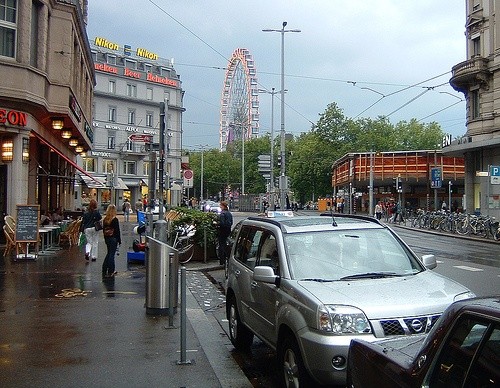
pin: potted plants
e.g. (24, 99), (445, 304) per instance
(165, 206), (218, 260)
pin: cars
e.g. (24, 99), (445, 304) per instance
(145, 200), (167, 215)
(199, 200), (229, 213)
(345, 293), (500, 388)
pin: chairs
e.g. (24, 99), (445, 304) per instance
(3, 216), (21, 256)
(58, 216), (83, 247)
(260, 238), (277, 258)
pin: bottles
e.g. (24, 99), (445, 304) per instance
(140, 232), (146, 244)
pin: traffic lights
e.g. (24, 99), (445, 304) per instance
(398, 181), (403, 193)
(166, 175), (174, 190)
(392, 178), (399, 192)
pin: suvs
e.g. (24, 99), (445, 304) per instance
(224, 209), (488, 388)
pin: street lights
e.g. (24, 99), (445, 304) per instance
(258, 21), (302, 209)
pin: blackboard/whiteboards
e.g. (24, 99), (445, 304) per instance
(14, 204), (41, 243)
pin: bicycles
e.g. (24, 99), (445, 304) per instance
(410, 208), (500, 241)
(166, 227), (196, 264)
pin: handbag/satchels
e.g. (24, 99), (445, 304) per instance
(374, 214), (377, 218)
(122, 203), (126, 211)
(78, 232), (82, 246)
(94, 221), (103, 231)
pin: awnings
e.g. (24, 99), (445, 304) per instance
(81, 175), (129, 190)
(142, 178), (182, 191)
(32, 129), (106, 188)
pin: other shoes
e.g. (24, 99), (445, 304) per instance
(92, 259), (96, 261)
(102, 274), (113, 281)
(85, 253), (89, 262)
(108, 271), (119, 275)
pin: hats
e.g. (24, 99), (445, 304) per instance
(221, 202), (228, 206)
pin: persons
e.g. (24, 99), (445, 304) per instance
(182, 195), (199, 209)
(83, 200), (102, 262)
(134, 195), (148, 222)
(102, 204), (121, 278)
(375, 199), (446, 224)
(218, 201), (233, 264)
(263, 194), (344, 215)
(122, 199), (130, 222)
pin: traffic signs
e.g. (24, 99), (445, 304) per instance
(128, 134), (153, 143)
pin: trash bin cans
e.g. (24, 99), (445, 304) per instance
(137, 210), (145, 226)
(145, 236), (180, 315)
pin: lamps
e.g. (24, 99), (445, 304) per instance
(61, 128), (72, 139)
(68, 136), (79, 146)
(22, 138), (30, 164)
(50, 116), (64, 129)
(2, 136), (14, 163)
(81, 149), (88, 156)
(74, 144), (84, 154)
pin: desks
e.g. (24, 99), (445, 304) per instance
(30, 224), (63, 255)
